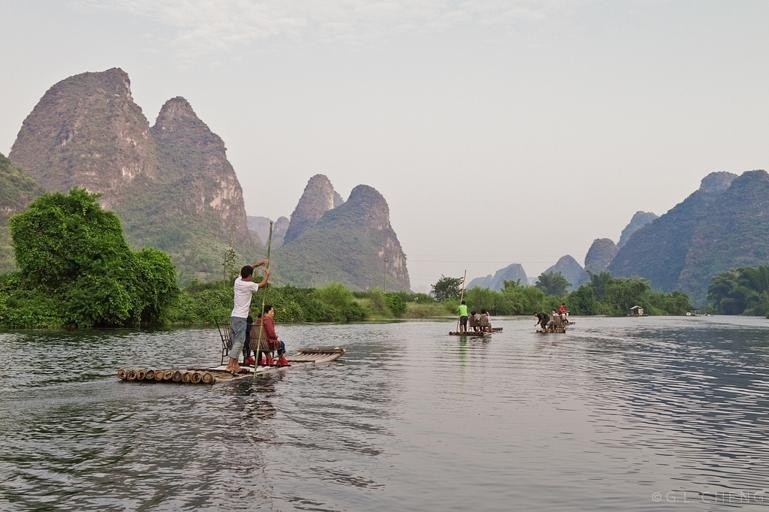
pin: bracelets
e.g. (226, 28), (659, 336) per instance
(254, 262), (259, 267)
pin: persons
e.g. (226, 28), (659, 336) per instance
(456, 300), (469, 333)
(224, 313), (252, 366)
(226, 257), (271, 373)
(546, 309), (560, 331)
(253, 306), (288, 366)
(481, 310), (493, 332)
(558, 303), (570, 321)
(533, 311), (550, 334)
(468, 310), (483, 333)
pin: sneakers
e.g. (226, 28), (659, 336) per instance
(244, 357), (286, 366)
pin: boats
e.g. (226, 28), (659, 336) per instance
(449, 327), (503, 338)
(117, 345), (346, 387)
(536, 321), (576, 333)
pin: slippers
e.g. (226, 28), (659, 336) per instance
(235, 368), (250, 374)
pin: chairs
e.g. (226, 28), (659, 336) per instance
(218, 323), (282, 369)
